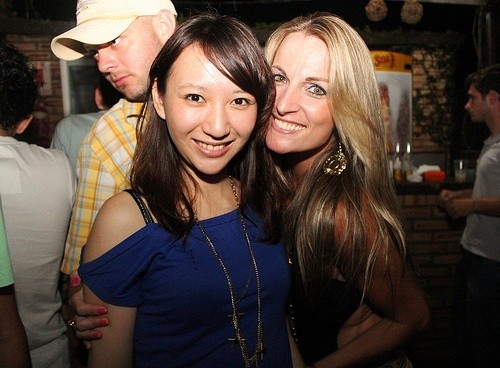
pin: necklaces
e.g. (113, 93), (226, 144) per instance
(187, 174), (264, 365)
(289, 296), (300, 348)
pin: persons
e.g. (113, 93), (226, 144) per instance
(0, 44), (77, 368)
(78, 13), (303, 368)
(67, 13), (430, 368)
(50, 0), (177, 322)
(439, 71), (500, 368)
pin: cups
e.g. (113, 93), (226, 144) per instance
(453, 160), (469, 183)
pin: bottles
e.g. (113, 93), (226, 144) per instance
(394, 157), (401, 181)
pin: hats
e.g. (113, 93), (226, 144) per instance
(50, 0), (178, 62)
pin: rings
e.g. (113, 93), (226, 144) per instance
(68, 320), (76, 331)
